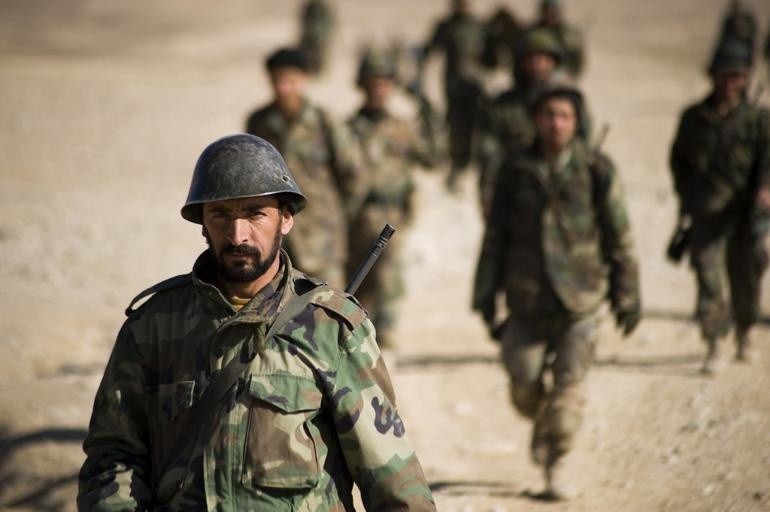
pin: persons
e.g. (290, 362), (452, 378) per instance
(77, 134), (438, 512)
(417, 0), (642, 502)
(664, 2), (770, 376)
(247, 1), (448, 507)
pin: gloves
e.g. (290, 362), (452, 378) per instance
(614, 304), (640, 339)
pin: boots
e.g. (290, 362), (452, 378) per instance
(736, 326), (758, 363)
(701, 336), (734, 377)
(543, 455), (580, 501)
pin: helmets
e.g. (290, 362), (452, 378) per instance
(526, 76), (584, 118)
(181, 133), (308, 226)
